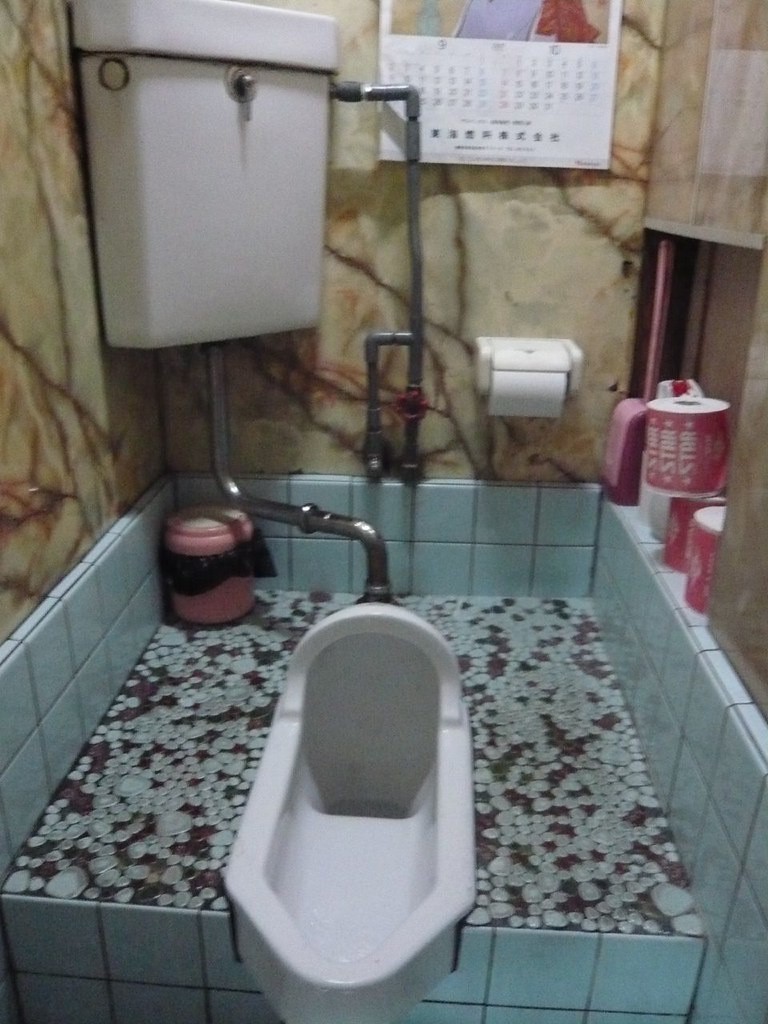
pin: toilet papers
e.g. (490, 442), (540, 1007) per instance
(643, 394), (727, 615)
(488, 369), (570, 421)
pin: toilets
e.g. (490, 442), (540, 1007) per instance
(225, 597), (478, 1024)
